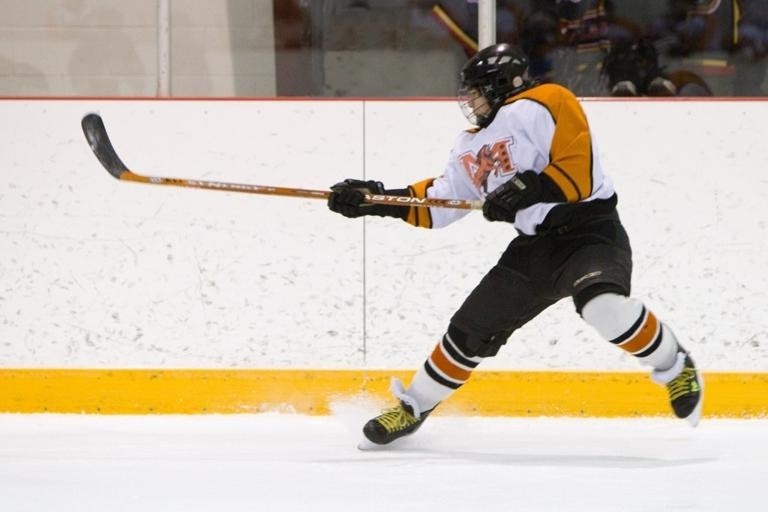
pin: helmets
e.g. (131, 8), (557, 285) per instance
(456, 43), (533, 128)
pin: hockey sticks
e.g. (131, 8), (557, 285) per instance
(81, 113), (484, 210)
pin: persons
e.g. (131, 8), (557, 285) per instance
(462, 0), (768, 98)
(327, 42), (701, 445)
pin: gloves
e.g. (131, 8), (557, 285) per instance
(328, 179), (411, 221)
(482, 172), (566, 223)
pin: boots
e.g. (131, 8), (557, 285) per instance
(362, 376), (441, 443)
(652, 344), (701, 419)
